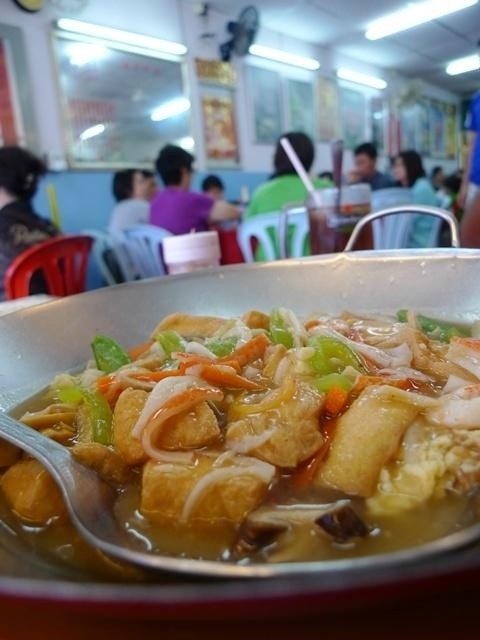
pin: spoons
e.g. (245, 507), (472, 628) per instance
(0, 409), (479, 580)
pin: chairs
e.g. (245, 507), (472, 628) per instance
(5, 233), (94, 300)
(124, 223), (175, 277)
(94, 223), (132, 285)
(371, 189), (414, 251)
(234, 205), (313, 262)
(428, 194), (452, 248)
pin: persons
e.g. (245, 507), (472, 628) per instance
(0, 146), (63, 302)
(348, 143), (393, 190)
(442, 89), (480, 248)
(150, 145), (244, 235)
(203, 176), (223, 200)
(391, 150), (442, 248)
(432, 167), (443, 190)
(108, 169), (160, 236)
(240, 132), (337, 262)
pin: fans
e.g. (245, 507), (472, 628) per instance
(218, 6), (259, 61)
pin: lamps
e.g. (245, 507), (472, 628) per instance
(248, 44), (320, 70)
(337, 69), (387, 90)
(361, 0), (479, 41)
(444, 54), (480, 76)
(55, 18), (189, 56)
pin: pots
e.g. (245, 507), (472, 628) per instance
(0, 204), (480, 618)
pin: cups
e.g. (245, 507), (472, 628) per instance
(306, 183), (374, 256)
(160, 231), (220, 276)
